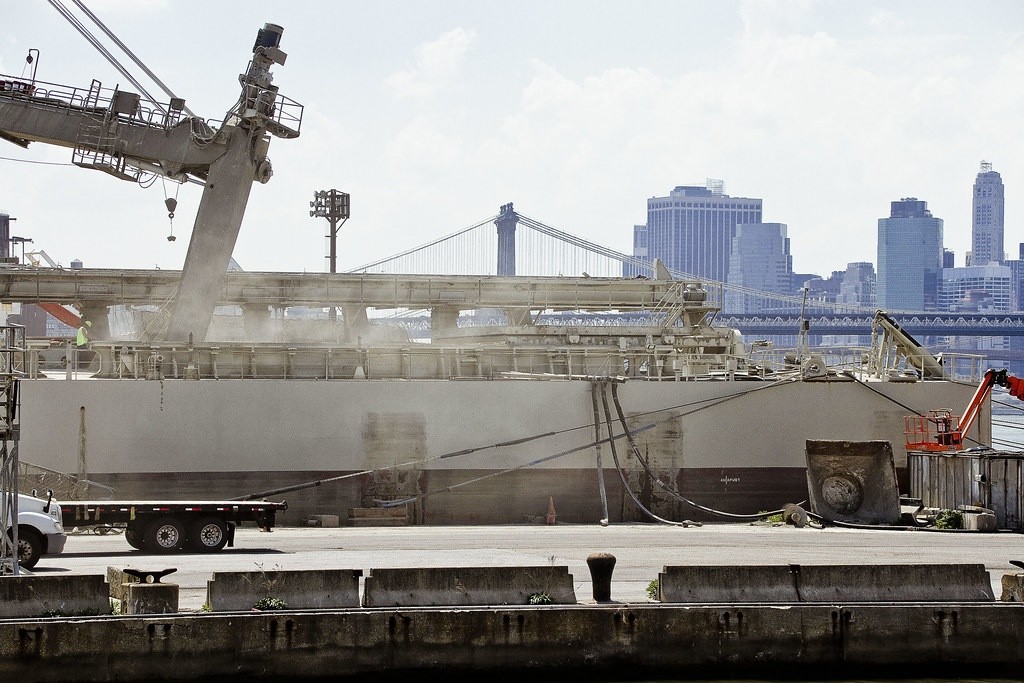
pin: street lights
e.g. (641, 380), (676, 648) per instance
(799, 287), (810, 323)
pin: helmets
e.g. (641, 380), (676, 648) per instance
(85, 321), (91, 327)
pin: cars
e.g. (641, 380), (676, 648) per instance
(0, 486), (70, 572)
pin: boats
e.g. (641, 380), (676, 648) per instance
(1, 2), (994, 523)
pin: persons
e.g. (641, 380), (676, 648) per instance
(76, 320), (93, 369)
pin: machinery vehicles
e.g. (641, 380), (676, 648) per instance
(901, 366), (1023, 451)
